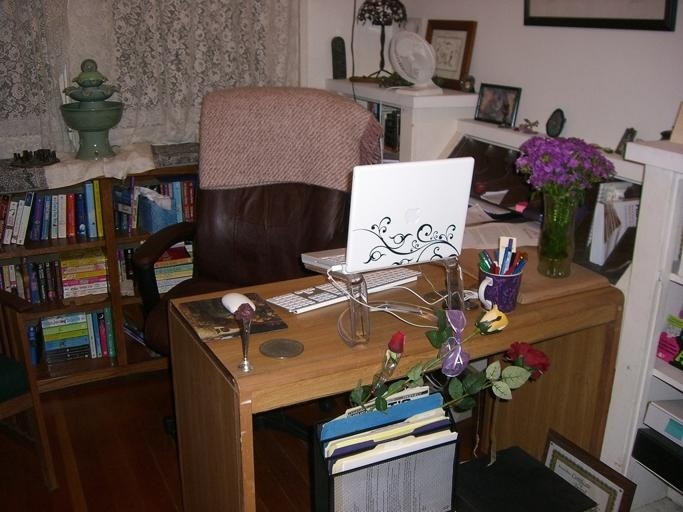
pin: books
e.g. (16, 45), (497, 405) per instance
(586, 183), (640, 266)
(0, 179), (195, 363)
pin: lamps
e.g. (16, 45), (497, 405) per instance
(350, 0), (408, 84)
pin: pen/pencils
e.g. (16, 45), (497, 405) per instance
(475, 239), (529, 293)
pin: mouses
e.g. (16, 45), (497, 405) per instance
(220, 291), (257, 318)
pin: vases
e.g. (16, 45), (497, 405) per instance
(536, 193), (576, 279)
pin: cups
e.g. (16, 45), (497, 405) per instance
(478, 262), (522, 314)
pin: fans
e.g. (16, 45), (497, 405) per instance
(388, 29), (444, 96)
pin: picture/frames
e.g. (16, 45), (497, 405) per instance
(524, 0), (678, 31)
(475, 82), (522, 128)
(425, 19), (478, 91)
(542, 427), (637, 512)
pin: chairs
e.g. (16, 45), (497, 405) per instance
(130, 86), (385, 443)
(0, 288), (60, 491)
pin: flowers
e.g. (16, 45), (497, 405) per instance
(515, 133), (618, 277)
(441, 341), (550, 410)
(348, 304), (509, 415)
(348, 329), (407, 412)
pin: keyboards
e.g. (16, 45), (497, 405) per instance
(266, 267), (421, 314)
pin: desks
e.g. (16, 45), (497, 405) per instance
(166, 245), (625, 512)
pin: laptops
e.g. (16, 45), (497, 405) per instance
(301, 155), (476, 278)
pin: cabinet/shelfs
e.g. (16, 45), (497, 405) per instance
(326, 79), (479, 163)
(0, 142), (198, 396)
(600, 141), (682, 512)
